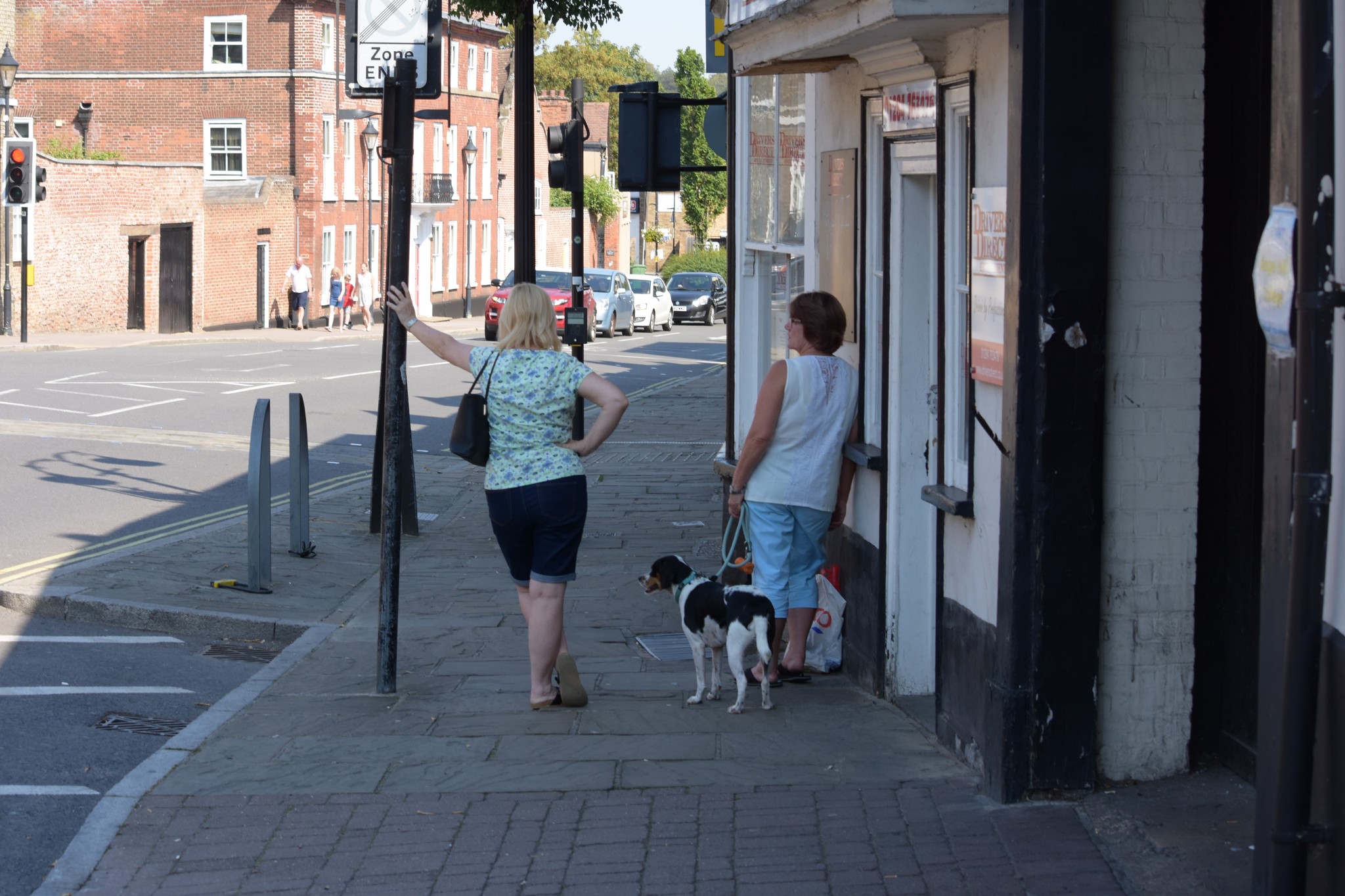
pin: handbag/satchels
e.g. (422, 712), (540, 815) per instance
(343, 287), (347, 296)
(782, 574), (847, 673)
(449, 347), (503, 467)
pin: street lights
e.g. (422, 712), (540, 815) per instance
(461, 135), (478, 319)
(361, 117), (380, 325)
(0, 42), (19, 337)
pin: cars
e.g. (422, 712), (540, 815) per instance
(571, 269), (636, 336)
(665, 272), (729, 326)
(484, 269), (597, 343)
(607, 275), (673, 333)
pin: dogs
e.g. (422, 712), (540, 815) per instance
(636, 553), (778, 715)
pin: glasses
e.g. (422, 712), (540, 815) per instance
(789, 318), (802, 327)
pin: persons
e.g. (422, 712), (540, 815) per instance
(384, 280), (629, 705)
(728, 289), (858, 686)
(281, 256), (375, 332)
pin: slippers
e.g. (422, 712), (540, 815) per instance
(776, 663), (811, 681)
(734, 667), (783, 687)
(530, 686), (564, 709)
(554, 652), (588, 707)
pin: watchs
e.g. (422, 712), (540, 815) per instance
(406, 317), (417, 331)
(729, 484), (745, 494)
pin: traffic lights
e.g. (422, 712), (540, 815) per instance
(2, 137), (36, 208)
(36, 165), (46, 204)
(546, 119), (576, 191)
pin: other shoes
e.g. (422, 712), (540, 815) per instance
(340, 327), (344, 333)
(348, 322), (353, 329)
(342, 325), (349, 330)
(325, 326), (332, 332)
(367, 324), (371, 331)
(362, 328), (367, 331)
(294, 325), (304, 331)
